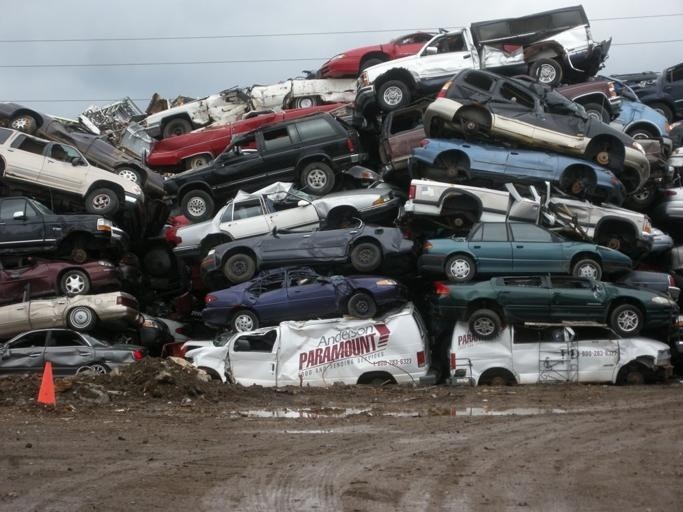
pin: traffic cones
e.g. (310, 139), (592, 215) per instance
(37, 362), (56, 407)
